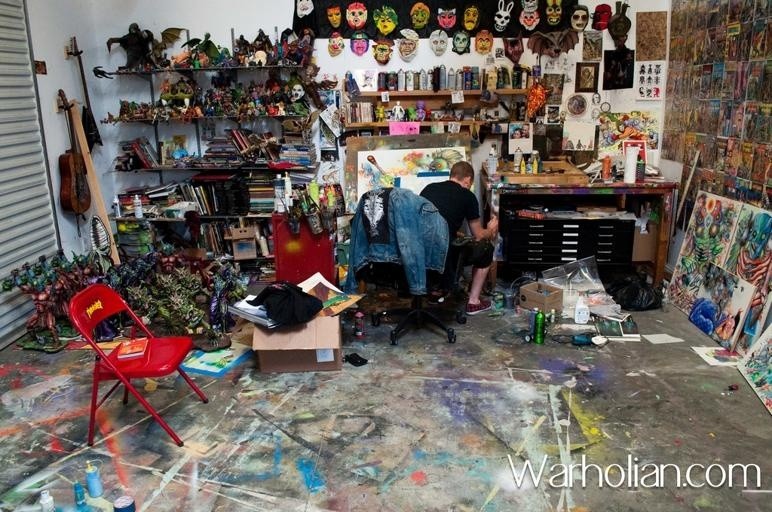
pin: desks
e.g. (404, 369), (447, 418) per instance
(479, 170), (681, 290)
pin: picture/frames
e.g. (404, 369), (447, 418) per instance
(575, 62), (599, 92)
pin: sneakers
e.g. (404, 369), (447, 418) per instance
(428, 288), (451, 304)
(465, 300), (491, 315)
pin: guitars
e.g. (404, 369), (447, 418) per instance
(58, 89), (90, 215)
(70, 36), (103, 153)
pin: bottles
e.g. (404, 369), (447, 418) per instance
(575, 291), (590, 324)
(521, 146), (542, 173)
(354, 312), (365, 337)
(488, 144), (498, 179)
(636, 159), (646, 181)
(602, 155), (611, 178)
(310, 180), (335, 207)
(519, 102), (526, 120)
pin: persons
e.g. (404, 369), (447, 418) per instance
(611, 120), (657, 149)
(3, 239), (248, 354)
(512, 124), (529, 139)
(93, 23), (338, 126)
(420, 162), (497, 315)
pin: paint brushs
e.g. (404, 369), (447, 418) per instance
(295, 187), (322, 214)
(277, 191), (290, 218)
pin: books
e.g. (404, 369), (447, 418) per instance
(117, 337), (148, 359)
(118, 128), (316, 286)
(346, 101), (376, 124)
(576, 206), (627, 217)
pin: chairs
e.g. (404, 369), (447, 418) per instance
(68, 285), (209, 447)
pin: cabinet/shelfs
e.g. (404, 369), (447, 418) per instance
(336, 90), (553, 155)
(110, 64), (323, 281)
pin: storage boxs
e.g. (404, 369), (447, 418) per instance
(252, 314), (343, 372)
(520, 281), (563, 315)
